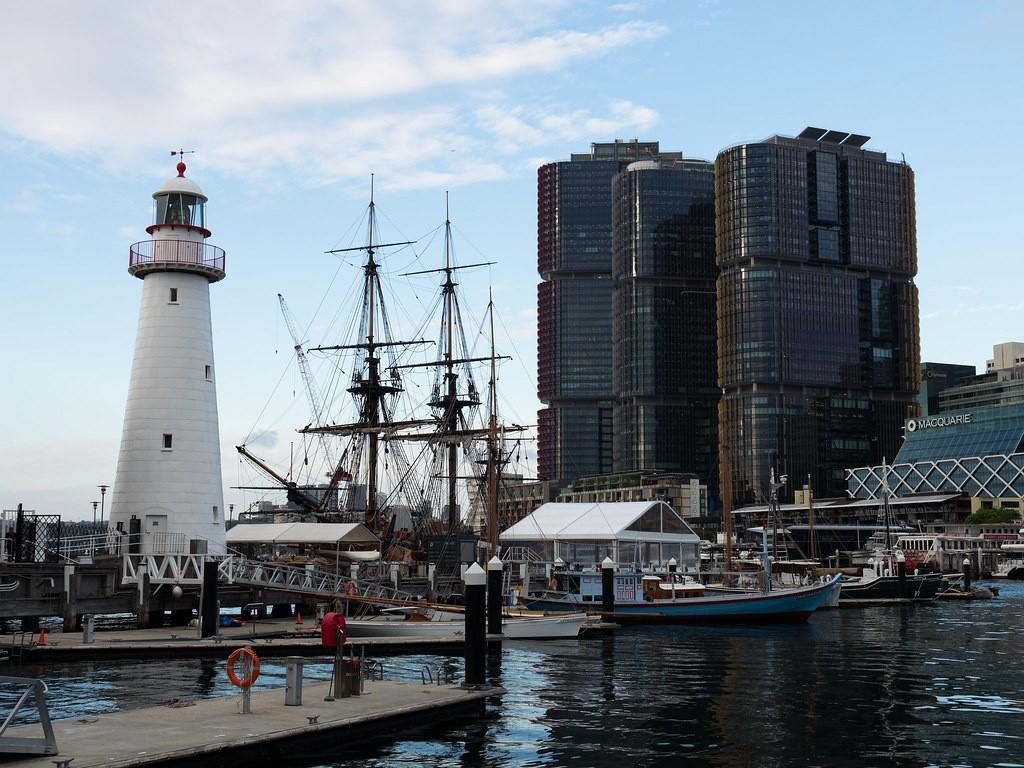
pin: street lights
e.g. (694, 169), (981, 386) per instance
(91, 501), (100, 523)
(97, 485), (110, 521)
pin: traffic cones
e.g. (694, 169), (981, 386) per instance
(36, 629), (47, 646)
(296, 612), (304, 625)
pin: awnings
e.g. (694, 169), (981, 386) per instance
(729, 493), (963, 515)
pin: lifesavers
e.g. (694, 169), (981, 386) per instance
(346, 582), (356, 595)
(644, 595), (653, 602)
(551, 579), (557, 590)
(226, 648), (260, 688)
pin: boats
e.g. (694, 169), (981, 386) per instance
(499, 498), (843, 628)
(705, 457), (942, 607)
(341, 611), (585, 644)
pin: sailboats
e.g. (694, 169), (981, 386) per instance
(241, 175), (539, 601)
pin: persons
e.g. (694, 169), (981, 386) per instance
(5, 526), (17, 562)
